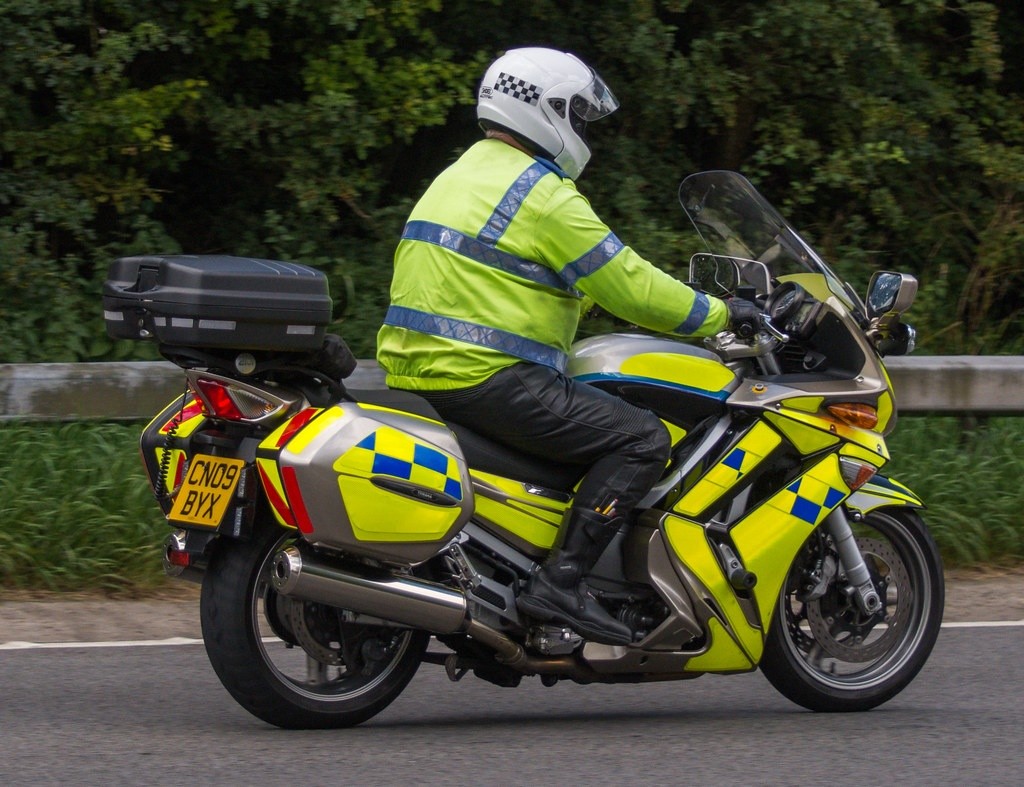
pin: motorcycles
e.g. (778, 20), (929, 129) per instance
(100, 170), (947, 730)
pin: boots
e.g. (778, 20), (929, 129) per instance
(515, 505), (633, 646)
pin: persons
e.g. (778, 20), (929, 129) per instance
(377, 48), (761, 645)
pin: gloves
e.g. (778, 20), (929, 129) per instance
(724, 296), (765, 341)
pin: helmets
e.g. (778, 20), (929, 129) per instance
(475, 47), (593, 183)
